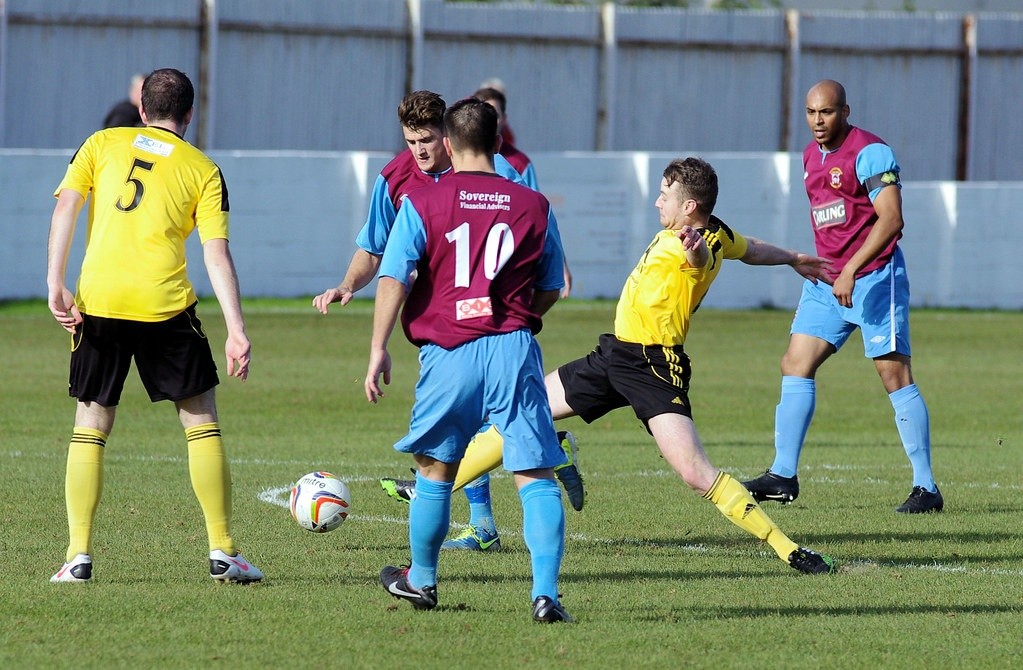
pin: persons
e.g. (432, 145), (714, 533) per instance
(380, 157), (834, 573)
(46, 67), (263, 588)
(103, 73), (147, 127)
(743, 79), (943, 516)
(313, 79), (594, 622)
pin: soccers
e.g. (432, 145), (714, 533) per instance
(288, 471), (352, 533)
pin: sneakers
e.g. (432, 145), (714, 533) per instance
(50, 554), (92, 583)
(210, 550), (263, 586)
(896, 484), (943, 513)
(381, 557), (438, 612)
(531, 594), (573, 624)
(553, 430), (584, 511)
(739, 469), (799, 502)
(380, 467), (418, 505)
(788, 548), (833, 576)
(439, 524), (502, 553)
